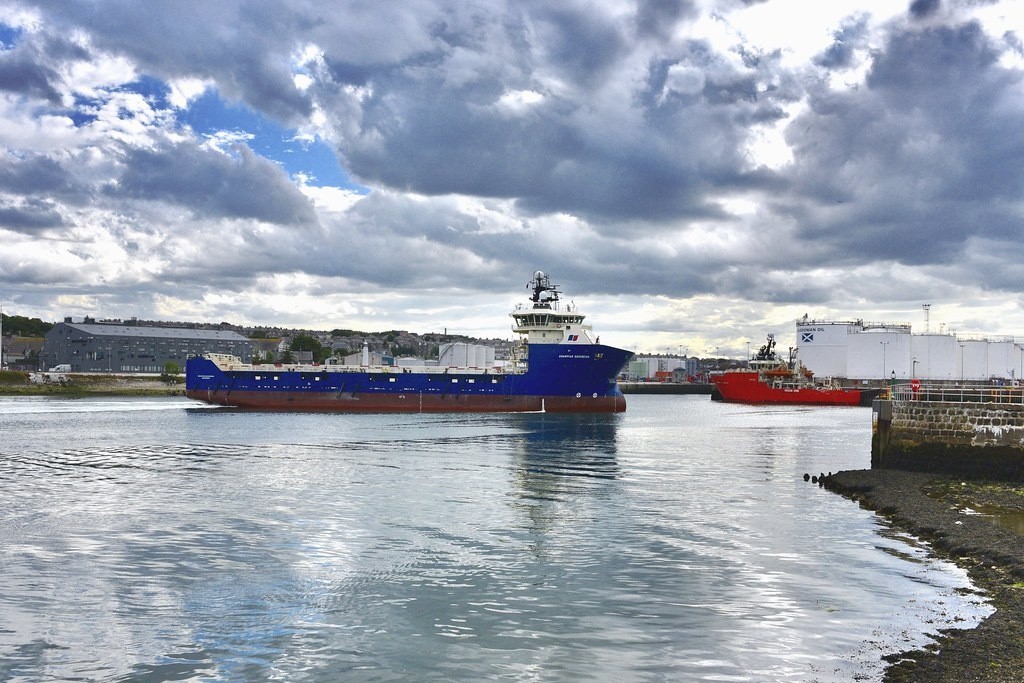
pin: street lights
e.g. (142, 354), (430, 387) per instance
(685, 347), (689, 358)
(746, 341), (752, 369)
(678, 344), (684, 359)
(667, 347), (670, 358)
(880, 340), (890, 390)
(715, 346), (720, 373)
(913, 361), (917, 382)
(959, 344), (967, 401)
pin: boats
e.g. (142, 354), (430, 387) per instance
(704, 332), (863, 406)
(184, 268), (636, 414)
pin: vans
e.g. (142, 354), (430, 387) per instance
(49, 363), (71, 374)
